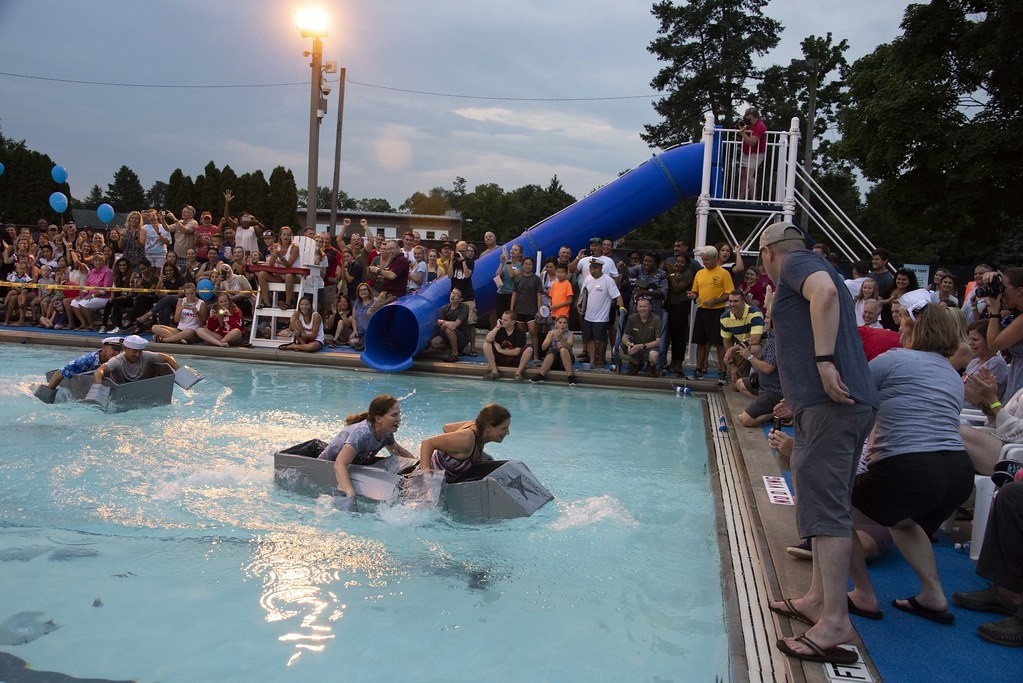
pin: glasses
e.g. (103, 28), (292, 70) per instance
(209, 246), (218, 250)
(907, 300), (931, 321)
(638, 296), (648, 300)
(451, 291), (461, 298)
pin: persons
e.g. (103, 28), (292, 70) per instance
(739, 107), (766, 204)
(0, 189), (477, 362)
(48, 335), (180, 390)
(479, 232), (793, 428)
(317, 395), (512, 496)
(756, 222), (1022, 663)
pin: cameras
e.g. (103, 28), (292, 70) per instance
(739, 118), (752, 127)
(975, 275), (1004, 299)
(453, 252), (460, 259)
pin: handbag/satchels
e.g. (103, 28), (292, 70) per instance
(493, 276), (503, 287)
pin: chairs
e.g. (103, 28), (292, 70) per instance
(939, 408), (1001, 560)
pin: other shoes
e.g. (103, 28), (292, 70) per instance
(786, 538), (812, 558)
(441, 349), (727, 385)
(953, 585), (1019, 615)
(4, 300), (363, 352)
(977, 613), (1023, 645)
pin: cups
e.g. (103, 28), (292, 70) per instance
(225, 247), (231, 258)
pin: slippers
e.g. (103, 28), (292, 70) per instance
(769, 599), (815, 626)
(846, 595), (884, 620)
(776, 633), (859, 664)
(893, 596), (955, 623)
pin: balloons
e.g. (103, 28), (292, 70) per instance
(197, 279), (215, 302)
(51, 165), (68, 184)
(49, 192), (68, 213)
(97, 203), (114, 224)
(0, 163), (4, 175)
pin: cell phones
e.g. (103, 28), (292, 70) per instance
(773, 416), (782, 433)
(242, 217), (251, 221)
(225, 247), (231, 254)
(134, 272), (140, 280)
(162, 211), (166, 216)
(503, 246), (507, 254)
(186, 304), (194, 309)
(213, 308), (225, 314)
(222, 271), (227, 281)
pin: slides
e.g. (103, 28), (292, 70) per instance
(361, 143), (708, 372)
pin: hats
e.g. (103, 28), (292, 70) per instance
(260, 230), (275, 239)
(47, 224), (59, 233)
(441, 242), (453, 250)
(760, 222), (805, 256)
(101, 337), (124, 350)
(200, 212), (211, 220)
(589, 258), (604, 267)
(590, 237), (602, 246)
(183, 204), (196, 214)
(124, 335), (148, 350)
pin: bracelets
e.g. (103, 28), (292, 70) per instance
(643, 344), (646, 350)
(377, 268), (381, 274)
(92, 294), (96, 298)
(299, 332), (301, 337)
(120, 236), (124, 239)
(133, 237), (138, 240)
(747, 354), (753, 361)
(990, 401), (1001, 410)
(280, 256), (284, 260)
(496, 325), (500, 330)
(989, 313), (1001, 318)
(506, 261), (512, 264)
(814, 355), (835, 362)
(174, 219), (178, 222)
(157, 232), (161, 235)
